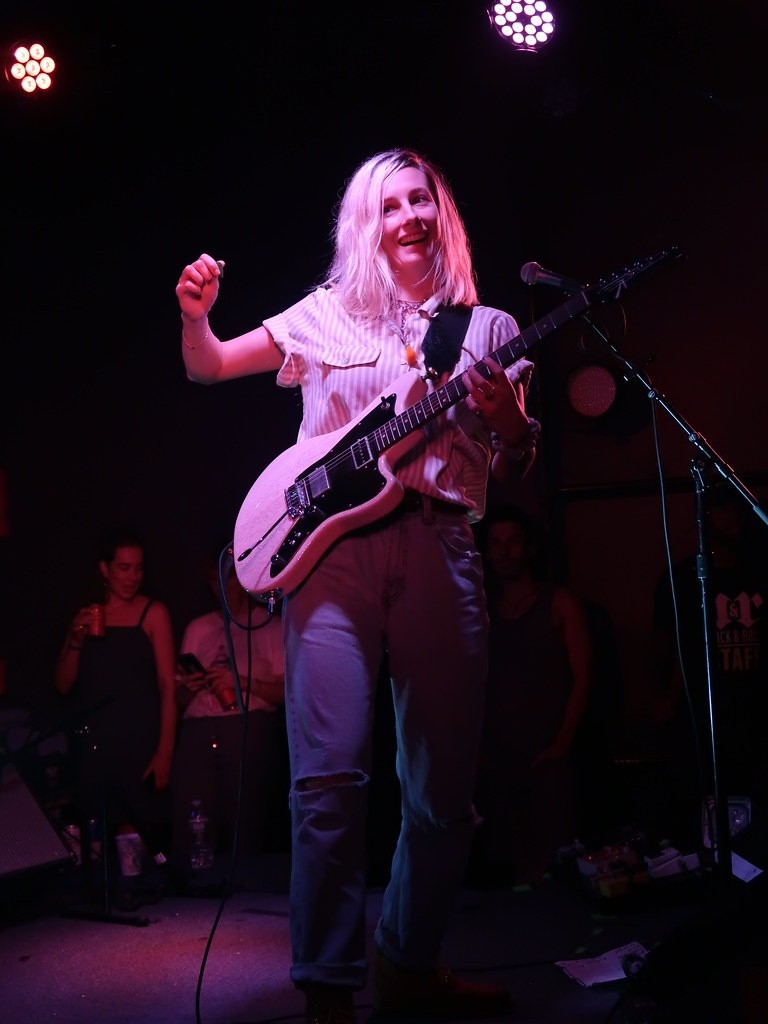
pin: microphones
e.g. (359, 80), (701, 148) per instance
(522, 262), (621, 304)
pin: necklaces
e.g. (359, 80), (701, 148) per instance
(387, 294), (428, 334)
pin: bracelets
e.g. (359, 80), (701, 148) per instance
(61, 637), (78, 650)
(182, 317), (209, 349)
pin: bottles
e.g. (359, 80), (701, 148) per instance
(188, 799), (209, 850)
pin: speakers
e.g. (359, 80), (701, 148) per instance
(0, 747), (79, 931)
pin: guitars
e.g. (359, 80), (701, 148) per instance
(232, 243), (690, 605)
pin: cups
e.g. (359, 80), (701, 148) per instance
(66, 826), (82, 866)
(115, 834), (144, 877)
(208, 680), (237, 713)
(87, 604), (105, 638)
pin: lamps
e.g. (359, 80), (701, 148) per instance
(565, 361), (653, 432)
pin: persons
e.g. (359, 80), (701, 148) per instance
(55, 482), (768, 997)
(174, 149), (543, 1024)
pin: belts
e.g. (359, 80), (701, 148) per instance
(389, 486), (473, 519)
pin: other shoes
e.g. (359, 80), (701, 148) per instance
(377, 959), (449, 1024)
(298, 984), (359, 1024)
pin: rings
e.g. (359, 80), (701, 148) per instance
(486, 386), (497, 399)
